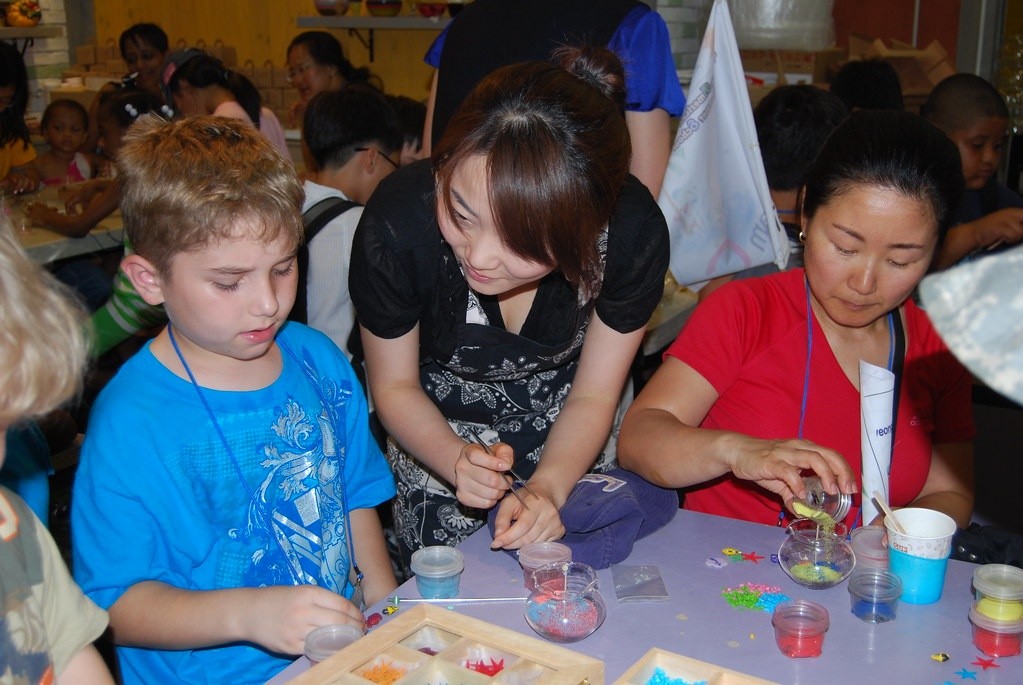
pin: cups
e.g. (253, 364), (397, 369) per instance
(848, 525), (890, 569)
(410, 546), (465, 599)
(795, 475), (851, 521)
(304, 622), (365, 665)
(972, 563), (1023, 602)
(969, 598), (1023, 657)
(882, 507), (957, 603)
(847, 569), (903, 624)
(772, 599), (829, 658)
(517, 541), (572, 591)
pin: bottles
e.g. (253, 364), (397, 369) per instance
(524, 562), (607, 644)
(778, 518), (857, 589)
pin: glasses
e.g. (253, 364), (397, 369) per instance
(285, 57), (317, 84)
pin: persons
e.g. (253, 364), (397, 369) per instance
(616, 135), (982, 543)
(1, 21), (425, 454)
(349, 38), (672, 588)
(696, 53), (905, 309)
(922, 71), (1023, 278)
(417, 1), (686, 204)
(0, 207), (116, 685)
(72, 113), (459, 685)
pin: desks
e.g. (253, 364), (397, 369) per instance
(1, 178), (128, 266)
(261, 505), (1023, 685)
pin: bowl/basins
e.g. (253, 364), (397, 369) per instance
(414, 2), (445, 17)
